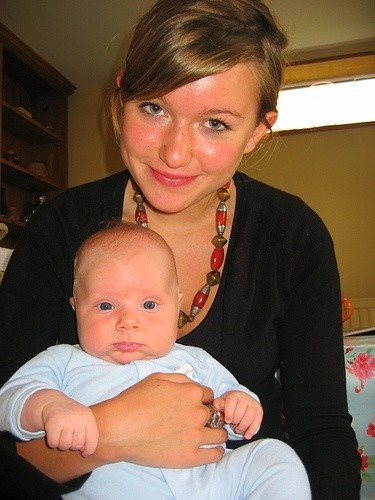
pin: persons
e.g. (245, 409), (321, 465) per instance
(0, 0), (362, 500)
(0, 219), (313, 500)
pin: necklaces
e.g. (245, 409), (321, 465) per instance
(132, 179), (232, 328)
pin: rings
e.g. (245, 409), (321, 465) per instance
(205, 403), (221, 430)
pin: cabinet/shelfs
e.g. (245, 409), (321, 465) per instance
(0, 22), (77, 247)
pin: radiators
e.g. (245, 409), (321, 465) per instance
(343, 298), (375, 332)
